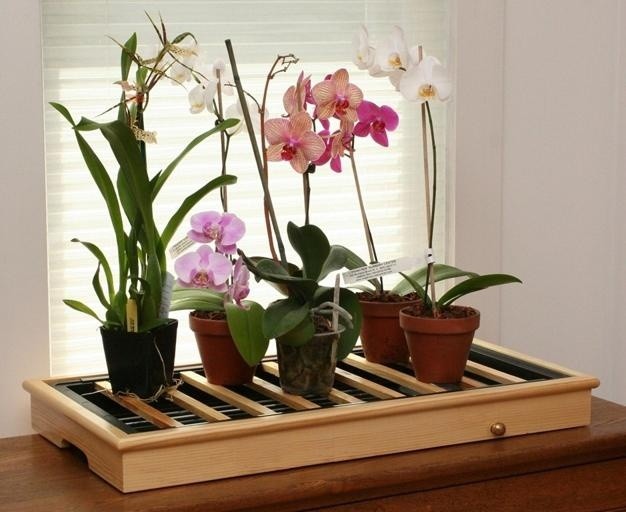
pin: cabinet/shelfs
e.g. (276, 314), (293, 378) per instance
(21, 337), (601, 495)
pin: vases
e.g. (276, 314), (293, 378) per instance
(355, 289), (424, 367)
(276, 320), (346, 398)
(188, 309), (255, 389)
(400, 301), (480, 387)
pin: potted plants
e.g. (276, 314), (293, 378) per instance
(47, 32), (240, 401)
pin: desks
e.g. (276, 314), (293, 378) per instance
(0, 397), (625, 511)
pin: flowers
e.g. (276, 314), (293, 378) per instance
(168, 46), (270, 369)
(310, 68), (399, 298)
(352, 20), (522, 319)
(223, 52), (364, 361)
